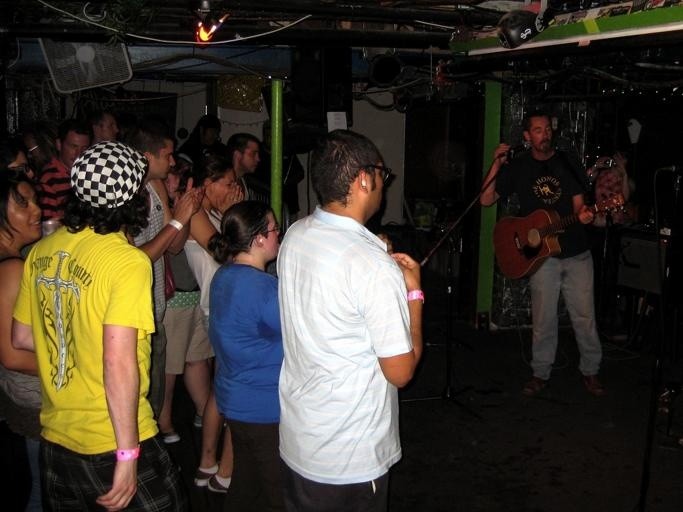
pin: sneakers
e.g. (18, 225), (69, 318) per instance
(163, 414), (203, 444)
(522, 377), (545, 394)
(193, 469), (229, 493)
(584, 376), (605, 396)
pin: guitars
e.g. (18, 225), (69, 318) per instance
(494, 193), (624, 279)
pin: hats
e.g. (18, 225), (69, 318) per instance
(71, 141), (148, 209)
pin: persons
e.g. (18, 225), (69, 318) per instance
(0, 110), (305, 510)
(477, 109), (607, 397)
(274, 128), (428, 510)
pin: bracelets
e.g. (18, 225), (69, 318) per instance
(407, 288), (424, 304)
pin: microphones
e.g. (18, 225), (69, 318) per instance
(671, 164), (683, 176)
(510, 142), (531, 158)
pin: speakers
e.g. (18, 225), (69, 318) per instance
(617, 229), (671, 294)
(399, 336), (459, 401)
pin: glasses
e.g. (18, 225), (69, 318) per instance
(358, 164), (391, 183)
